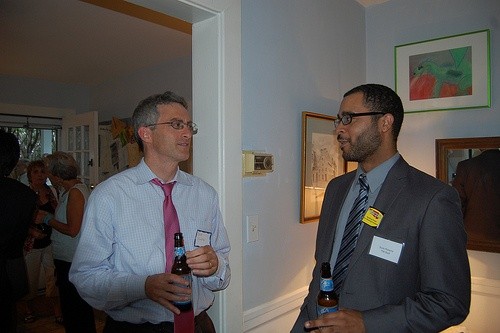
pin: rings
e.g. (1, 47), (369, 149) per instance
(208, 261), (212, 268)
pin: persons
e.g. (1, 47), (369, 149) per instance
(0, 129), (96, 333)
(69, 90), (231, 333)
(290, 83), (471, 333)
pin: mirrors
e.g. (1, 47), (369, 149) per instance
(435, 136), (500, 254)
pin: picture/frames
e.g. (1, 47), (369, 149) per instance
(394, 28), (492, 113)
(301, 111), (349, 224)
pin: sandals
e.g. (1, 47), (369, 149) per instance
(55, 315), (63, 324)
(23, 313), (33, 323)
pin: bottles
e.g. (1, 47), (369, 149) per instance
(37, 223), (52, 234)
(317, 262), (338, 319)
(171, 233), (193, 313)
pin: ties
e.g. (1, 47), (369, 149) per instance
(150, 177), (195, 332)
(333, 174), (369, 297)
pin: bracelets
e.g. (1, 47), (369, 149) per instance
(44, 214), (53, 225)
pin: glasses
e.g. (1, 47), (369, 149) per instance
(334, 111), (384, 130)
(145, 121), (198, 134)
